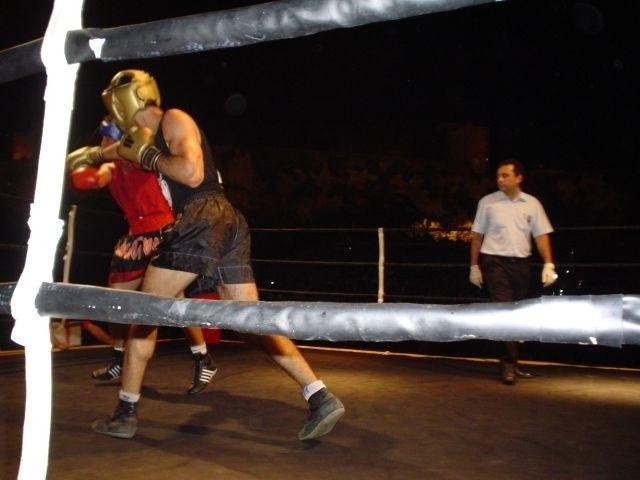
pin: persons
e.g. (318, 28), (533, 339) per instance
(470, 163), (561, 384)
(68, 69), (345, 440)
(73, 109), (219, 394)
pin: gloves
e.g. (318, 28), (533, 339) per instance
(468, 264), (485, 289)
(539, 263), (560, 288)
(115, 124), (164, 173)
(65, 143), (105, 177)
(66, 163), (102, 193)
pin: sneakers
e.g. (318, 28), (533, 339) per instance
(296, 386), (349, 444)
(500, 358), (535, 385)
(185, 351), (219, 395)
(89, 349), (125, 384)
(87, 398), (141, 440)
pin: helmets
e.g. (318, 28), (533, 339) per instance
(100, 67), (163, 134)
(98, 114), (123, 142)
